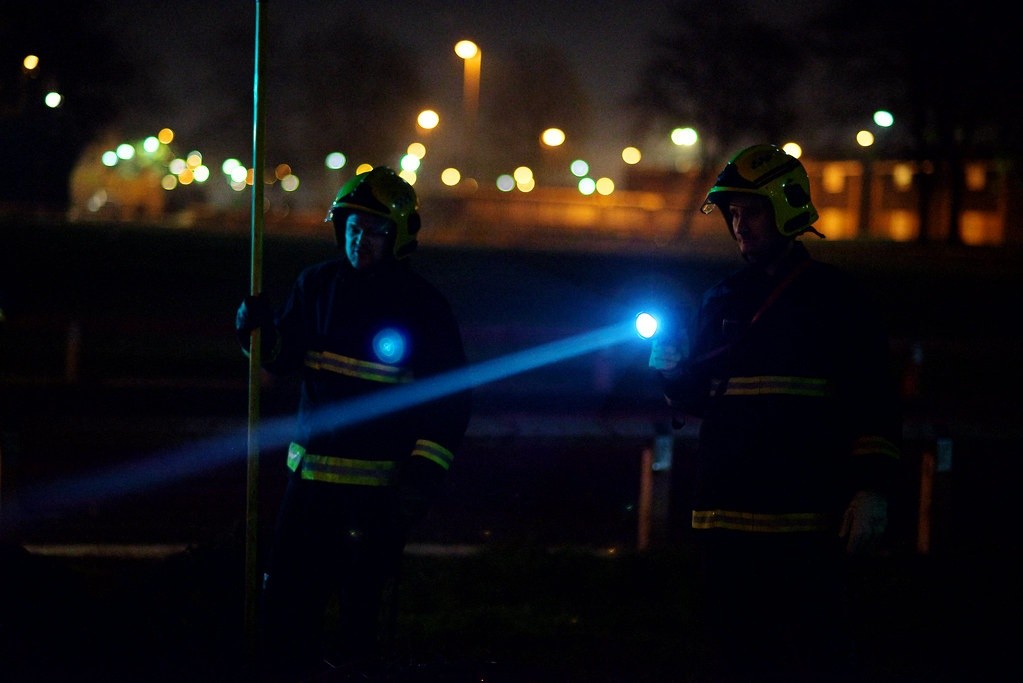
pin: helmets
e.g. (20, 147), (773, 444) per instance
(330, 166), (421, 261)
(699, 144), (819, 237)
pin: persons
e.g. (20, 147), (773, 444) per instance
(234, 166), (473, 683)
(629, 143), (902, 683)
